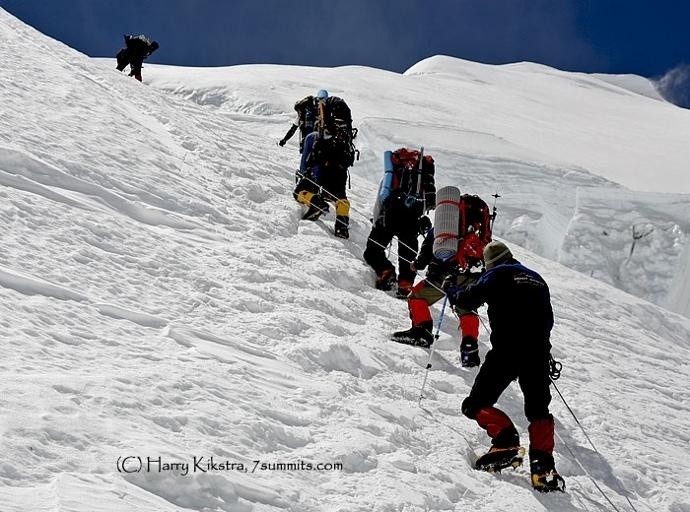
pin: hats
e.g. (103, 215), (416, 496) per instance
(482, 240), (513, 271)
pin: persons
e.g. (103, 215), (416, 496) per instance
(116, 33), (160, 82)
(279, 89), (355, 239)
(363, 147), (565, 493)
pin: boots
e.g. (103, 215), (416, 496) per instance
(527, 450), (560, 493)
(334, 215), (350, 239)
(391, 319), (434, 345)
(302, 195), (329, 221)
(375, 270), (418, 300)
(475, 428), (522, 471)
(461, 337), (479, 365)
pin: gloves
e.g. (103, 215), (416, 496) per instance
(440, 275), (455, 290)
(410, 260), (416, 270)
(279, 139), (286, 147)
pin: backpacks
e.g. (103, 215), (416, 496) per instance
(316, 96), (355, 168)
(390, 150), (436, 218)
(449, 193), (490, 269)
